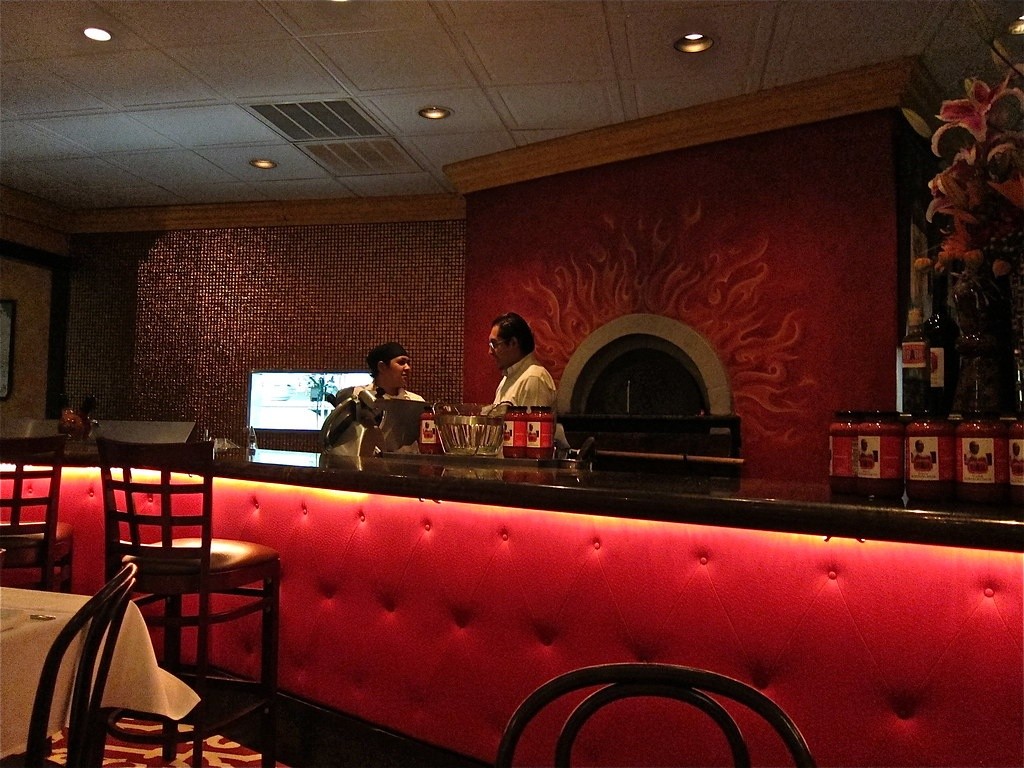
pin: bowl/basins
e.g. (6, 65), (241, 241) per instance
(432, 403), (507, 457)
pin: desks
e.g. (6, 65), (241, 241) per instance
(0, 585), (202, 768)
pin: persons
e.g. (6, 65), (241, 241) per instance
(337, 342), (425, 453)
(479, 311), (570, 451)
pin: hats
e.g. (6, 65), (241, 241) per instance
(366, 342), (409, 373)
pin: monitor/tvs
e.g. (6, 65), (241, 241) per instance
(247, 369), (374, 433)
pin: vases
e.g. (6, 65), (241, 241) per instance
(948, 262), (1020, 417)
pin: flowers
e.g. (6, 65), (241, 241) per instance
(898, 0), (1024, 280)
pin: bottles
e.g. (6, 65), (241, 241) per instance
(901, 306), (961, 417)
(248, 425), (258, 449)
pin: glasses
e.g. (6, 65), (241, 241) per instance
(489, 337), (514, 350)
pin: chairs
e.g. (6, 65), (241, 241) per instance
(0, 435), (74, 596)
(496, 662), (819, 767)
(0, 562), (137, 768)
(95, 436), (280, 768)
